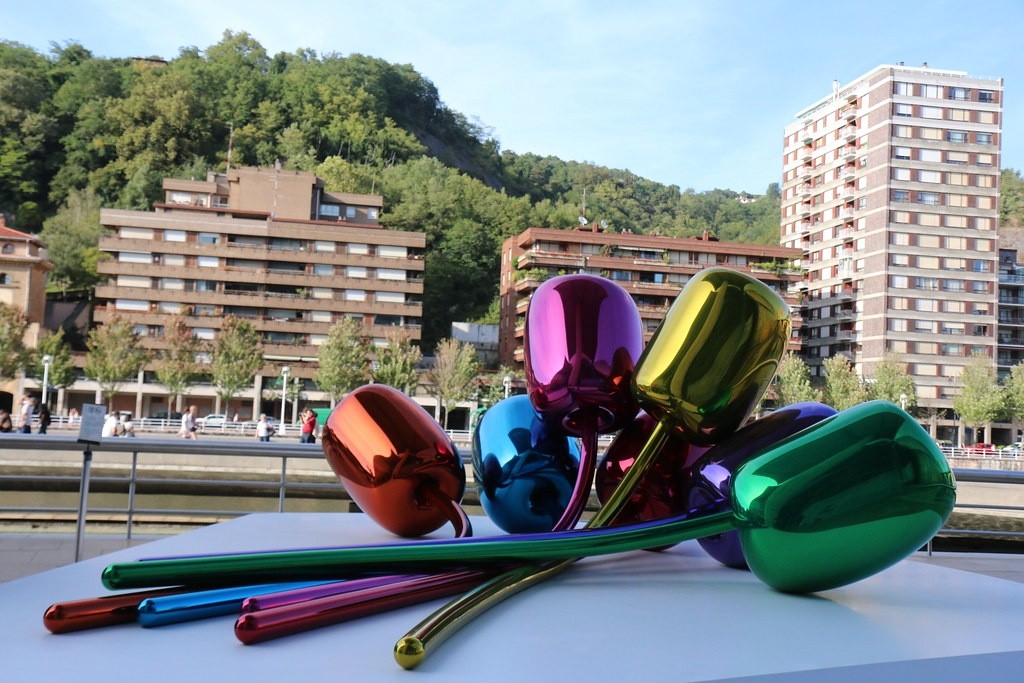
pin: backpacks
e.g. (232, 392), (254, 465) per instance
(40, 411), (52, 425)
(118, 423), (129, 436)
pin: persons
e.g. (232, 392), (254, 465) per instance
(0, 392), (136, 440)
(178, 405), (321, 444)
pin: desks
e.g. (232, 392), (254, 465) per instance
(0, 512), (1024, 683)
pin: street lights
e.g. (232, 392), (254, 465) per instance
(900, 393), (907, 411)
(279, 366), (291, 436)
(502, 376), (511, 399)
(40, 354), (51, 407)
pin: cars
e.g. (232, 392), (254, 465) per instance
(243, 416), (281, 424)
(195, 414), (230, 428)
(964, 443), (996, 455)
(143, 411), (182, 420)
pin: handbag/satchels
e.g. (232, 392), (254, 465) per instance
(266, 422), (275, 436)
(307, 435), (316, 444)
